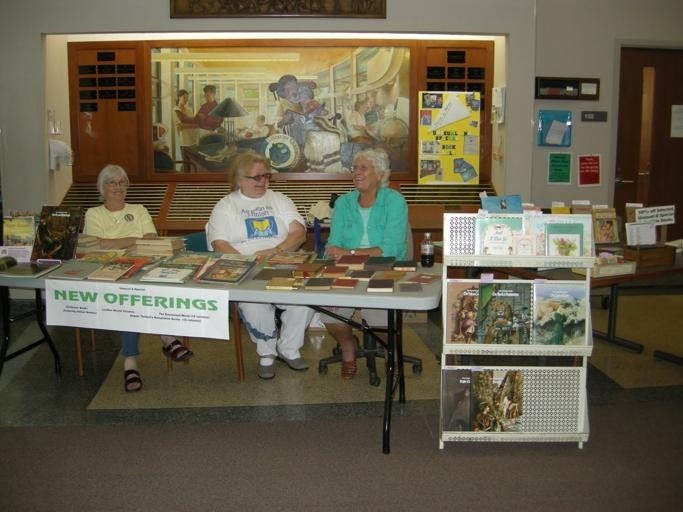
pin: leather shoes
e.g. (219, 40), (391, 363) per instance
(258, 355), (276, 380)
(277, 348), (309, 371)
(384, 342), (401, 378)
(342, 333), (360, 380)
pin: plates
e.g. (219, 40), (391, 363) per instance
(259, 134), (301, 172)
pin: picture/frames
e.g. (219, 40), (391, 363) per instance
(143, 40), (417, 183)
(170, 0), (386, 18)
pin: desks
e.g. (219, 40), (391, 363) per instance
(433, 240), (683, 361)
(0, 252), (442, 455)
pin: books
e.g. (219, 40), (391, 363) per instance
(0, 203), (82, 282)
(82, 235), (440, 292)
(442, 281), (588, 432)
(480, 194), (619, 256)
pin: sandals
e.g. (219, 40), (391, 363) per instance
(162, 339), (194, 362)
(124, 369), (143, 392)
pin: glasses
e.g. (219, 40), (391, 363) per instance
(245, 172), (272, 181)
(104, 180), (127, 187)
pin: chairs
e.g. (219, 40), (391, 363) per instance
(184, 232), (282, 382)
(319, 221), (422, 386)
(75, 224), (173, 376)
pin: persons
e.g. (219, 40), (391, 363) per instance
(433, 96), (442, 108)
(171, 75), (377, 172)
(320, 146), (408, 379)
(426, 160), (435, 175)
(205, 155), (313, 379)
(82, 164), (195, 391)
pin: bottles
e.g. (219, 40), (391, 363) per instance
(419, 231), (435, 268)
